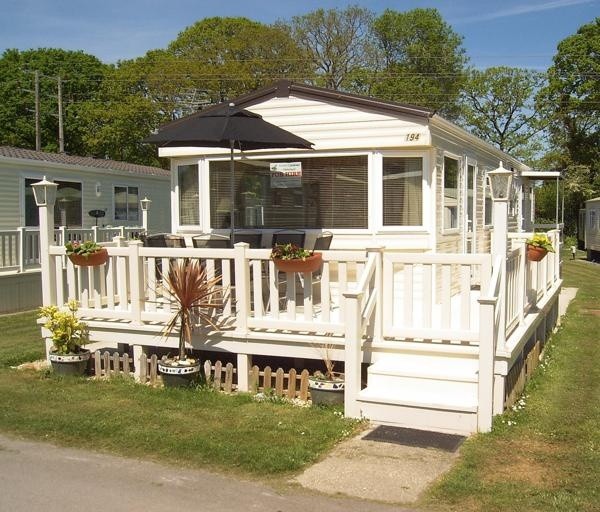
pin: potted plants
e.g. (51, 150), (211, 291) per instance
(525, 233), (556, 253)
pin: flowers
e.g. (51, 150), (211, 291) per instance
(271, 242), (313, 262)
(36, 298), (100, 355)
(65, 238), (108, 258)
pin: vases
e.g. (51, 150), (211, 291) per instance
(527, 245), (547, 261)
(69, 249), (108, 266)
(307, 375), (345, 408)
(273, 252), (322, 273)
(157, 357), (202, 388)
(49, 348), (90, 376)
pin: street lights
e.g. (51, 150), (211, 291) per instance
(140, 195), (153, 237)
(27, 173), (60, 369)
(486, 160), (515, 358)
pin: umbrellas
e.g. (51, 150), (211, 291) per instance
(138, 103), (315, 247)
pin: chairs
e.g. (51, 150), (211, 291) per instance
(229, 232), (263, 281)
(192, 235), (235, 286)
(266, 229), (306, 311)
(295, 230), (332, 319)
(145, 233), (189, 280)
